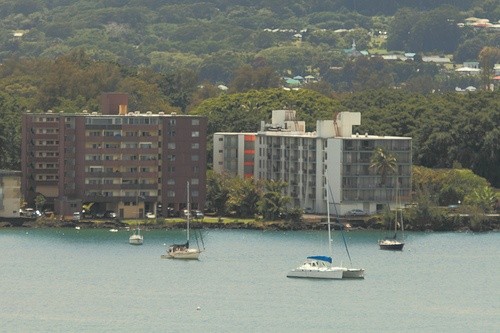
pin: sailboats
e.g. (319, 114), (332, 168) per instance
(286, 173), (364, 278)
(379, 162), (406, 249)
(129, 221), (144, 245)
(167, 181), (206, 261)
